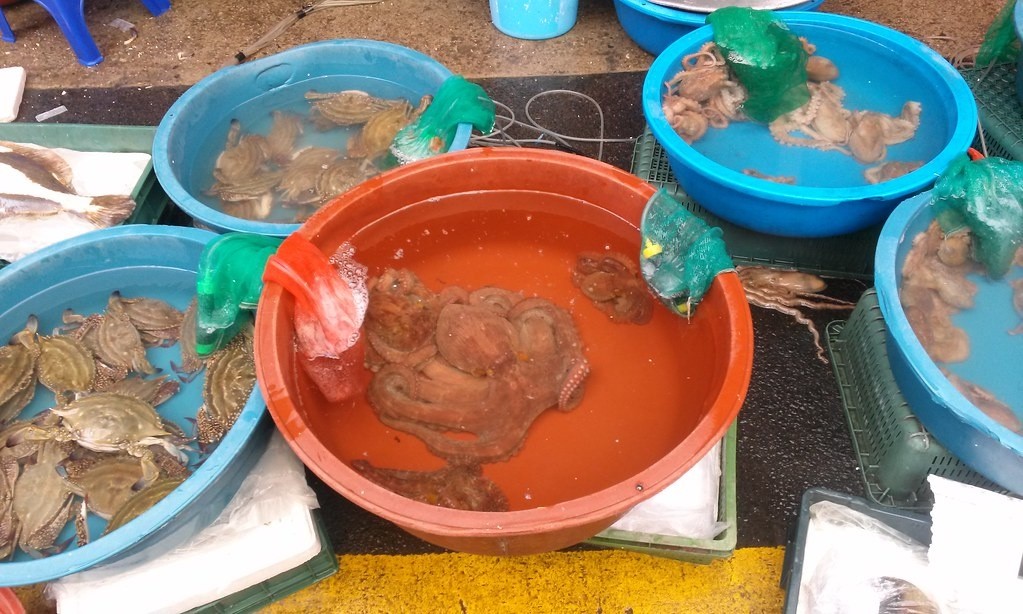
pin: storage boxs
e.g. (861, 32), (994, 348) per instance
(956, 60), (1023, 161)
(630, 123), (885, 280)
(180, 509), (339, 613)
(591, 416), (737, 565)
(780, 488), (933, 614)
(824, 288), (1023, 514)
(0, 122), (169, 267)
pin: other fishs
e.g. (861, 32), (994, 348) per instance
(0, 139), (138, 229)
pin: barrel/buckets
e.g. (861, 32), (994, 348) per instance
(491, 0), (580, 40)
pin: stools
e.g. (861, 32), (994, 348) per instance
(0, 0), (175, 68)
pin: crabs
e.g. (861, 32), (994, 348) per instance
(200, 78), (434, 222)
(0, 290), (259, 563)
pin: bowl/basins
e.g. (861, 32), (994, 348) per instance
(643, 9), (979, 239)
(153, 37), (473, 239)
(0, 224), (280, 590)
(613, 1), (828, 58)
(253, 145), (753, 557)
(874, 176), (1023, 498)
